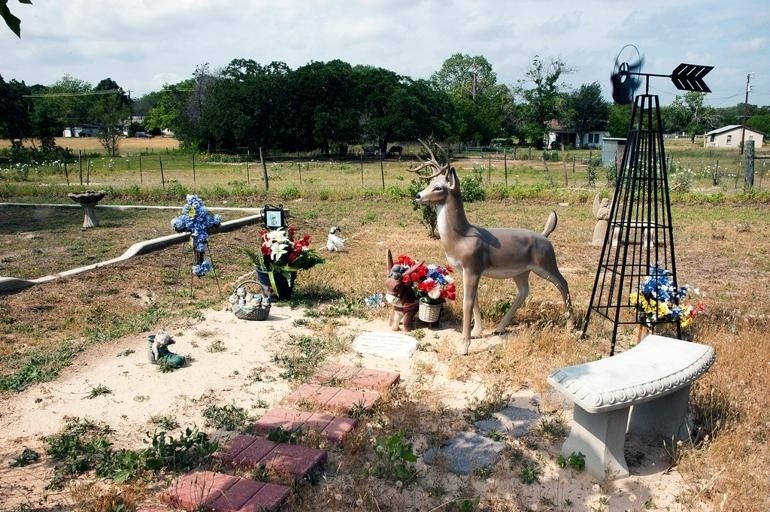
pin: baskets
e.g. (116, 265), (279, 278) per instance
(417, 296), (443, 323)
(231, 280), (272, 321)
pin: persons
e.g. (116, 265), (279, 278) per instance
(592, 197), (614, 246)
(326, 226), (349, 253)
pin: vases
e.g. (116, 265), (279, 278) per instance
(252, 266), (302, 303)
(418, 298), (445, 324)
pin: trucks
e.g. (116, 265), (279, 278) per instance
(135, 131), (155, 138)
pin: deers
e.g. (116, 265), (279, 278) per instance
(405, 136), (576, 357)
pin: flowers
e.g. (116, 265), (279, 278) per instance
(630, 264), (706, 331)
(244, 227), (324, 270)
(397, 253), (458, 300)
(171, 193), (221, 253)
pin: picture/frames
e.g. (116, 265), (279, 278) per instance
(264, 209), (285, 228)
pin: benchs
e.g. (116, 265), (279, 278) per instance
(547, 333), (718, 483)
(609, 219), (658, 248)
